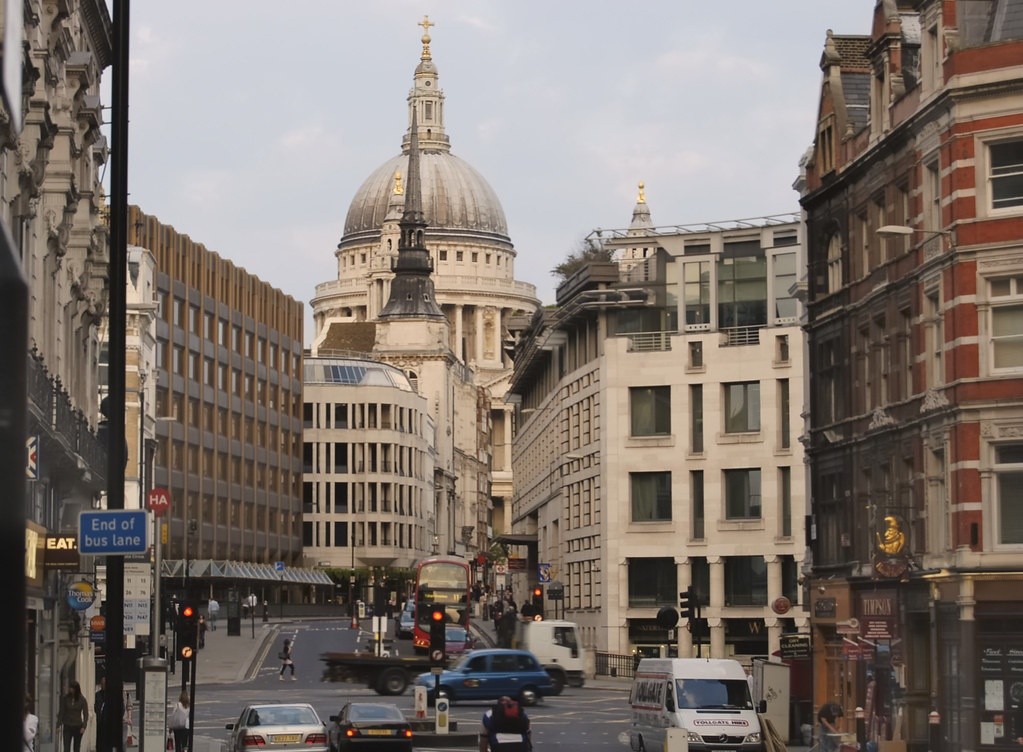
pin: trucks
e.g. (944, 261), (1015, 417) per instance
(318, 622), (477, 697)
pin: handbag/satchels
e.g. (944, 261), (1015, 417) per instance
(279, 652), (288, 659)
(168, 702), (185, 731)
(167, 734), (173, 750)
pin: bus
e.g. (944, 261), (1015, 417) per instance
(405, 555), (481, 656)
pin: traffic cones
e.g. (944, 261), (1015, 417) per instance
(349, 616), (360, 630)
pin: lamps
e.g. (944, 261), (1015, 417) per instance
(566, 454), (599, 459)
(877, 224), (950, 235)
(521, 408), (553, 413)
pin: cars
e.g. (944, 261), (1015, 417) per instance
(328, 702), (414, 752)
(226, 703), (331, 752)
(394, 599), (415, 641)
(411, 648), (559, 709)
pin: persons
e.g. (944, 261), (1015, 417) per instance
(477, 695), (535, 752)
(94, 676), (134, 752)
(817, 702), (845, 752)
(279, 639), (298, 681)
(240, 592), (258, 619)
(56, 681), (89, 752)
(1013, 693), (1023, 752)
(23, 697), (39, 752)
(198, 614), (207, 648)
(208, 597), (220, 631)
(469, 579), (539, 649)
(168, 690), (191, 752)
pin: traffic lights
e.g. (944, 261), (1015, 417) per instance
(680, 585), (696, 631)
(533, 584), (544, 622)
(177, 604), (198, 662)
(430, 603), (446, 668)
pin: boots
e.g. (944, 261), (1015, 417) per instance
(291, 675), (298, 680)
(279, 675), (284, 681)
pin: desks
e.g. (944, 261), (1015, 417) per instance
(823, 732), (848, 752)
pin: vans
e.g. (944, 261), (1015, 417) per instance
(629, 656), (768, 752)
(512, 618), (588, 688)
